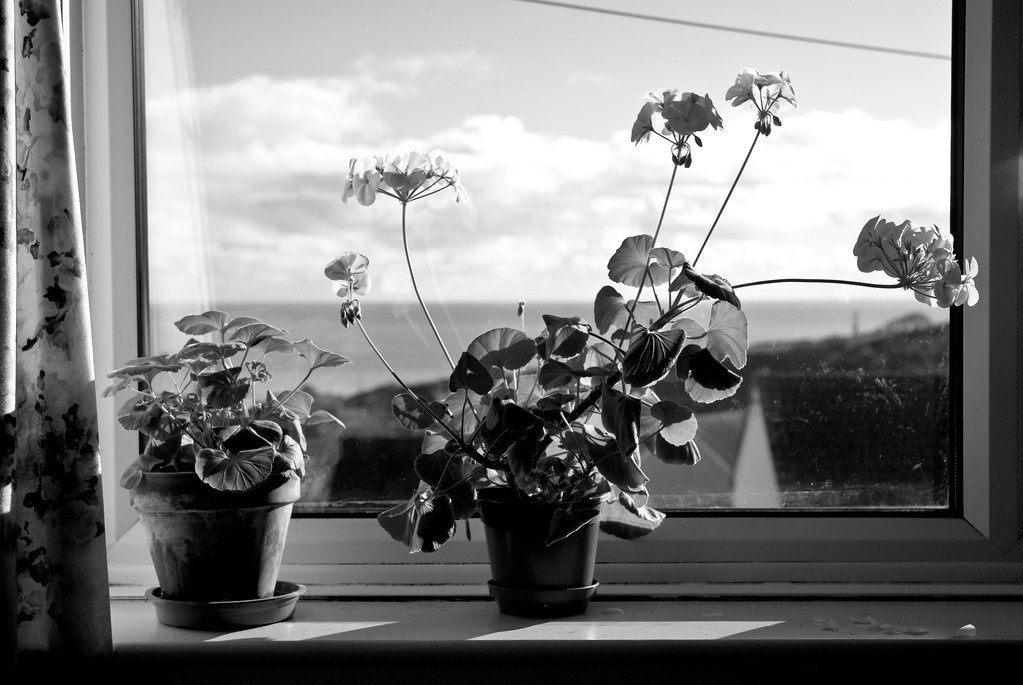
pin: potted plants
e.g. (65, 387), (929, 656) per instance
(102, 308), (353, 600)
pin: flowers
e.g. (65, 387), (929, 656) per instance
(325, 66), (980, 554)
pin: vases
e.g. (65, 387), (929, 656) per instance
(473, 484), (600, 617)
